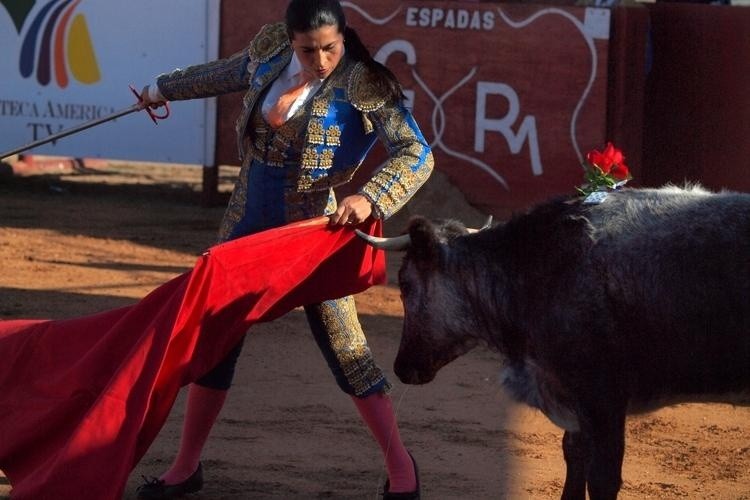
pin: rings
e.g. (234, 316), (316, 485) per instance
(346, 220), (353, 225)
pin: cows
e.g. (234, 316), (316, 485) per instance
(353, 186), (750, 500)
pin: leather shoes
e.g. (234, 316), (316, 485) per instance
(381, 448), (422, 500)
(136, 461), (204, 500)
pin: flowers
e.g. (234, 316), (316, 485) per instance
(568, 143), (631, 200)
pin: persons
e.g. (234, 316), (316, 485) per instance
(136, 0), (436, 499)
